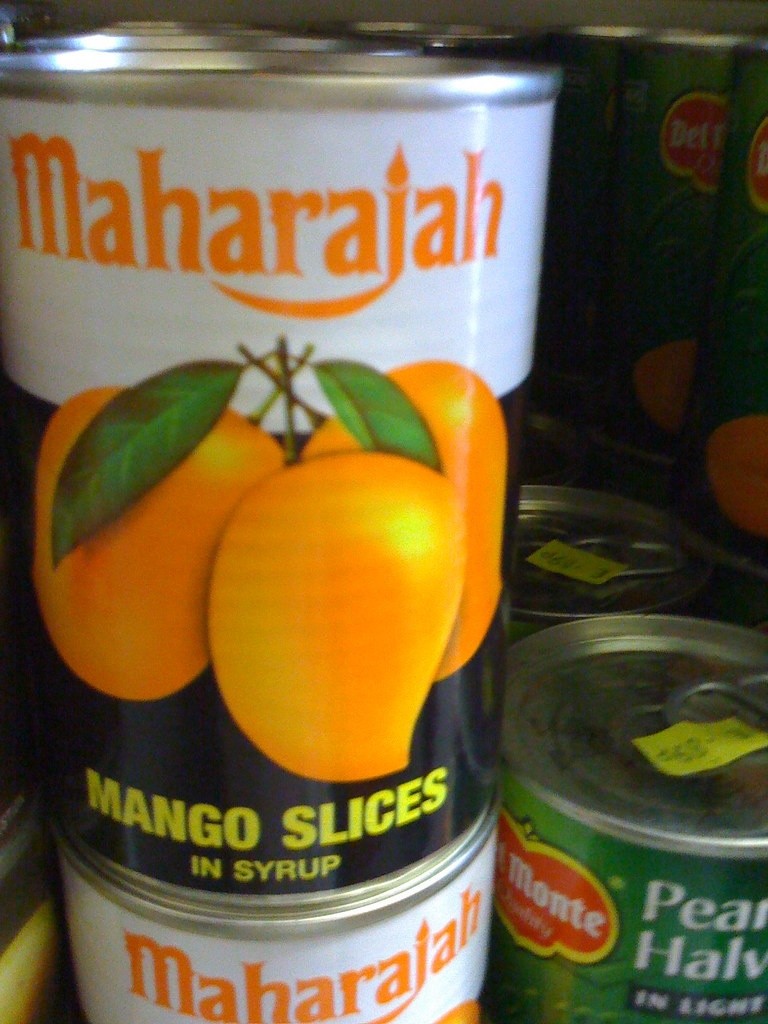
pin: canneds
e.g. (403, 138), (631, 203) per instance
(0, 16), (768, 1024)
